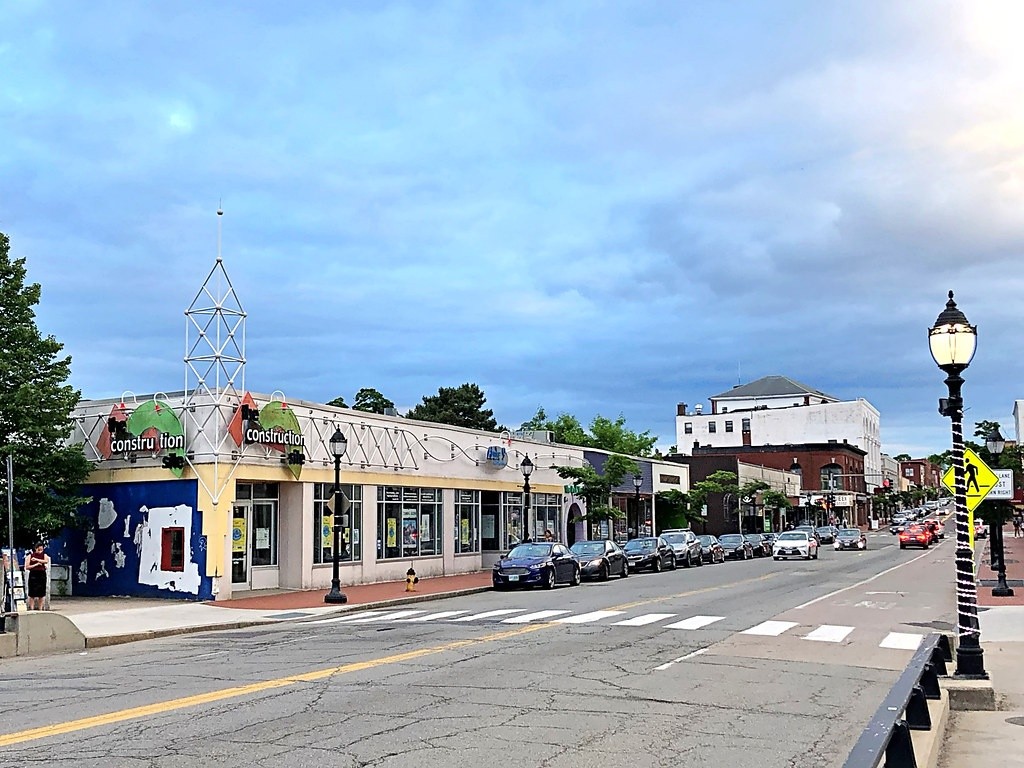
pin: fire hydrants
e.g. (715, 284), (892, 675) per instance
(405, 568), (419, 591)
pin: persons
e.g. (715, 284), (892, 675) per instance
(25, 543), (49, 611)
(842, 516), (848, 529)
(542, 530), (554, 542)
(834, 516), (840, 530)
(522, 533), (532, 543)
(1013, 518), (1022, 538)
(828, 515), (834, 526)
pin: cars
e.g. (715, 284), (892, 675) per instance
(744, 533), (781, 558)
(772, 530), (818, 560)
(623, 537), (676, 573)
(492, 542), (581, 590)
(570, 541), (628, 582)
(795, 526), (867, 551)
(890, 497), (951, 550)
(696, 535), (726, 564)
(718, 534), (753, 561)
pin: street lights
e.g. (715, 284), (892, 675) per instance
(751, 491), (758, 532)
(520, 453), (535, 543)
(928, 289), (991, 680)
(324, 423), (347, 604)
(985, 424), (1015, 597)
(807, 492), (812, 523)
(632, 473), (643, 538)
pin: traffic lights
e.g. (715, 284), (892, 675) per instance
(885, 478), (893, 493)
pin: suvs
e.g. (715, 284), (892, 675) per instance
(659, 529), (703, 569)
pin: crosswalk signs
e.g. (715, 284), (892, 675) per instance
(942, 447), (999, 512)
